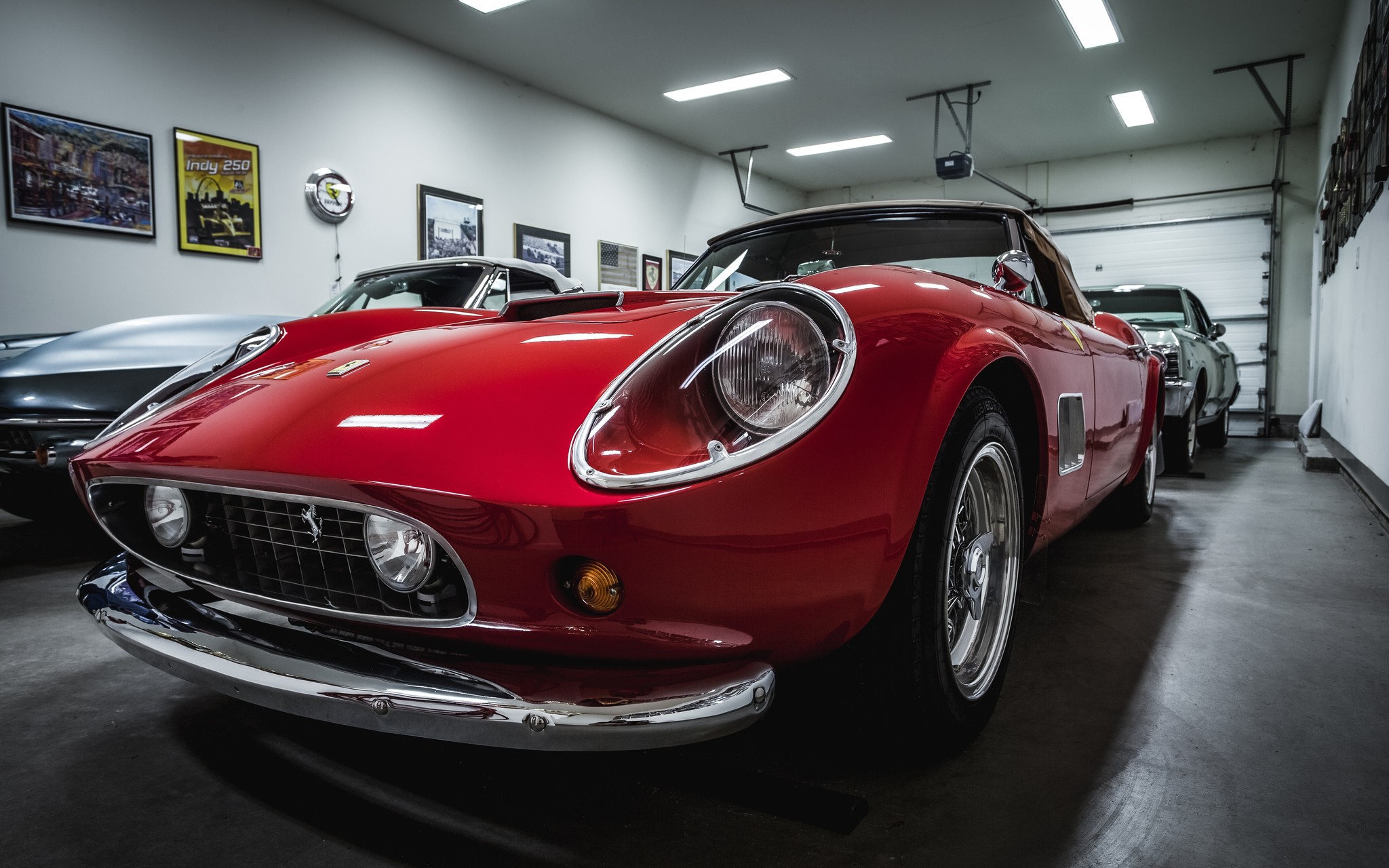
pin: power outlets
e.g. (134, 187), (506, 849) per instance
(330, 283), (338, 299)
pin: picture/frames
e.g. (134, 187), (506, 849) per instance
(513, 222), (572, 279)
(642, 254), (662, 291)
(597, 240), (639, 292)
(173, 127), (263, 260)
(1318, 0), (1389, 284)
(666, 249), (712, 290)
(725, 269), (761, 291)
(1, 101), (157, 238)
(416, 184), (485, 261)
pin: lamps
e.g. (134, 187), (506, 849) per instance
(933, 84), (975, 180)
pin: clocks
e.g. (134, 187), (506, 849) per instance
(305, 167), (355, 224)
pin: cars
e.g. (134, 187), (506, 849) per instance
(1, 253), (587, 525)
(1074, 283), (1242, 473)
(67, 196), (1165, 781)
